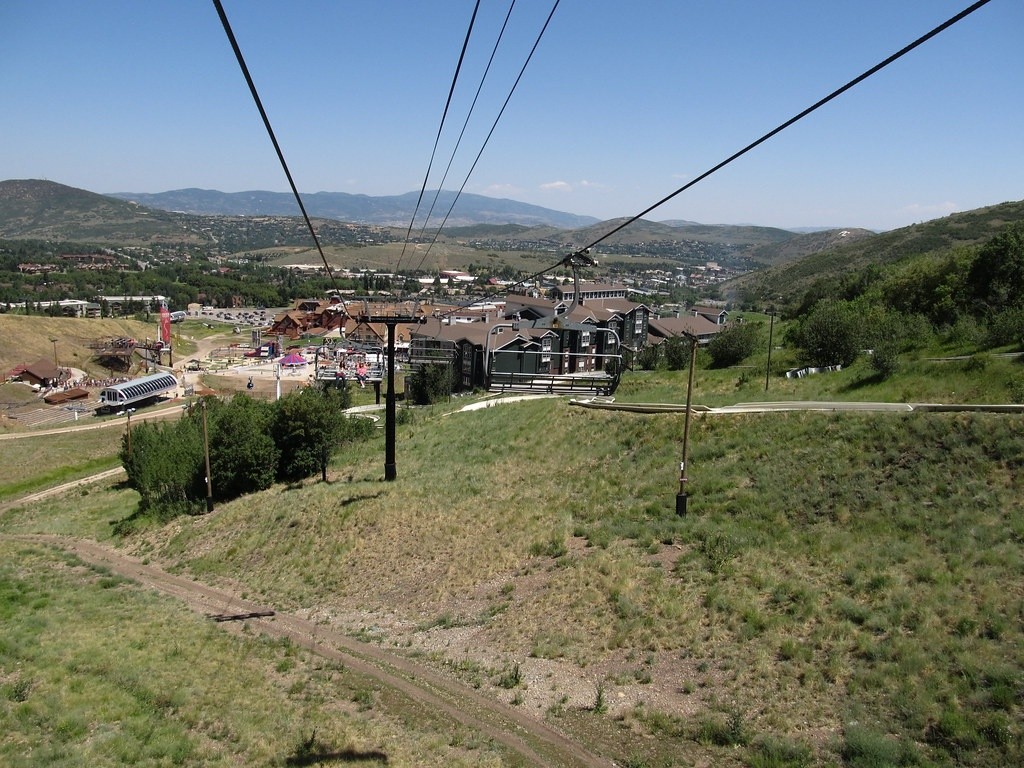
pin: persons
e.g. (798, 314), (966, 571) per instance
(356, 362), (367, 389)
(335, 361), (347, 391)
